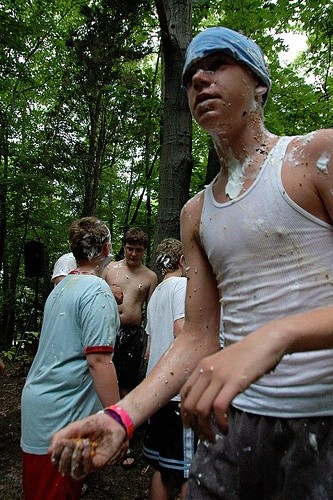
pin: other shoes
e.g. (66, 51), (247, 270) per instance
(123, 448), (134, 467)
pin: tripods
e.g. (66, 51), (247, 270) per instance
(12, 277), (42, 363)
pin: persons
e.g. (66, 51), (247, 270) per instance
(53, 212), (118, 290)
(19, 219), (123, 499)
(50, 26), (333, 499)
(102, 223), (160, 404)
(140, 231), (211, 496)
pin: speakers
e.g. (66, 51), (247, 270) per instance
(23, 240), (47, 277)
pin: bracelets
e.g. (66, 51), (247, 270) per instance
(101, 405), (139, 437)
